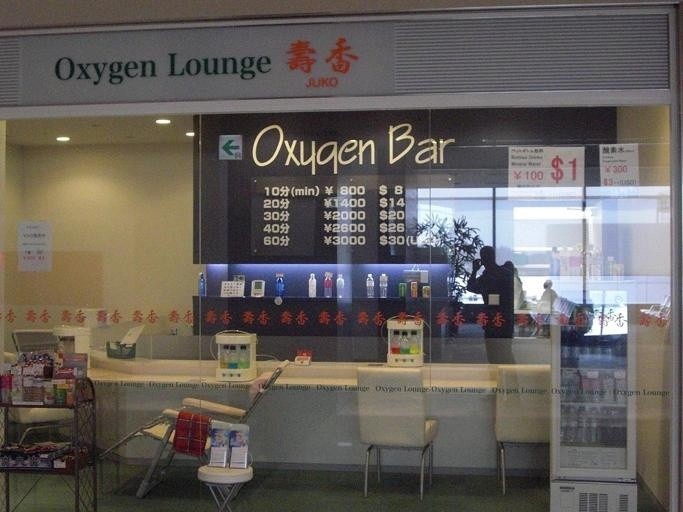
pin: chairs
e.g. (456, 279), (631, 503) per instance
(97, 358), (291, 499)
(353, 364), (440, 502)
(495, 364), (551, 497)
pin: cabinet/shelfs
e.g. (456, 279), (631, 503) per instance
(0, 373), (98, 512)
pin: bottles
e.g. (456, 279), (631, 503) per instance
(274, 271), (431, 300)
(391, 331), (420, 354)
(220, 344), (250, 368)
(551, 246), (625, 278)
(198, 271), (206, 297)
(561, 327), (626, 444)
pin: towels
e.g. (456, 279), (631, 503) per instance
(173, 410), (211, 457)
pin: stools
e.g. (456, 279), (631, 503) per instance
(194, 462), (253, 512)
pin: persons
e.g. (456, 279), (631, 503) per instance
(504, 260), (524, 311)
(466, 246), (520, 410)
(531, 279), (559, 307)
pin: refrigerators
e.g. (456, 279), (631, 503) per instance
(549, 278), (638, 512)
